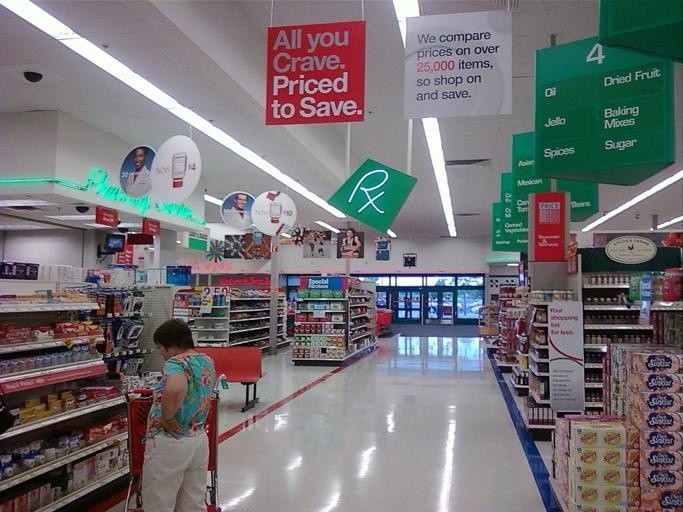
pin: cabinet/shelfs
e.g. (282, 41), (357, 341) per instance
(0, 286), (173, 510)
(292, 287), (377, 366)
(174, 286), (292, 353)
(477, 247), (682, 440)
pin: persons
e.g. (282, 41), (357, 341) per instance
(231, 194), (251, 228)
(307, 230), (327, 256)
(140, 317), (217, 512)
(340, 229), (362, 257)
(126, 148), (152, 196)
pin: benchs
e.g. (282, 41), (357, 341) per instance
(194, 347), (269, 413)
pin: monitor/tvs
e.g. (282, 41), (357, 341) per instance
(104, 233), (125, 252)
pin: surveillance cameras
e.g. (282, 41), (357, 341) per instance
(24, 72), (42, 82)
(118, 228), (128, 233)
(76, 206), (89, 213)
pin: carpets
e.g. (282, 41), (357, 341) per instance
(387, 322), (479, 337)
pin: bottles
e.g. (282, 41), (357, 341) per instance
(581, 271), (653, 344)
(583, 351), (604, 401)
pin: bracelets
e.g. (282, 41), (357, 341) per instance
(164, 416), (176, 424)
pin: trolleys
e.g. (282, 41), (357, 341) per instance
(121, 388), (223, 512)
